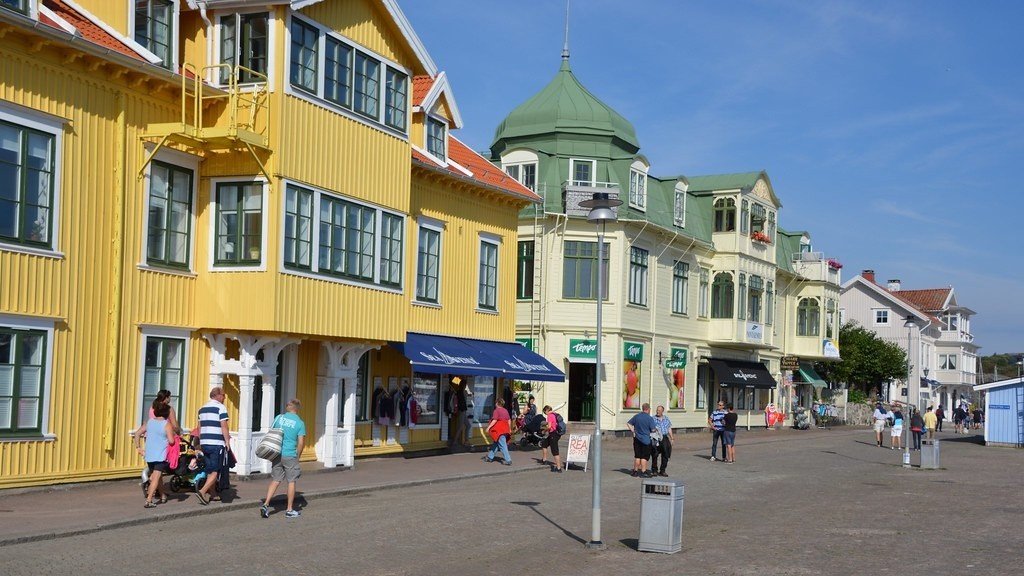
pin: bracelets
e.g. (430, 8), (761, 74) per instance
(671, 439), (674, 440)
(632, 432), (635, 433)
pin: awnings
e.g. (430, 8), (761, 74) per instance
(387, 331), (565, 382)
(793, 363), (827, 388)
(702, 358), (777, 388)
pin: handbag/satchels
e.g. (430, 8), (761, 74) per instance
(255, 427), (284, 461)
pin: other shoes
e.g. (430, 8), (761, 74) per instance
(286, 510), (301, 518)
(209, 495), (222, 501)
(160, 491), (167, 503)
(140, 482), (150, 497)
(195, 491), (207, 505)
(502, 458), (512, 465)
(486, 454), (492, 461)
(710, 456), (716, 461)
(261, 505), (269, 518)
(539, 458), (547, 464)
(891, 447), (901, 450)
(144, 501), (157, 507)
(719, 458), (726, 461)
(725, 460), (736, 464)
(630, 470), (638, 476)
(652, 472), (668, 477)
(555, 466), (561, 472)
(642, 473), (652, 477)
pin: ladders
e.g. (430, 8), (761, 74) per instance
(1016, 385), (1024, 447)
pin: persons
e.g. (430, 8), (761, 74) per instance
(627, 404), (656, 478)
(651, 406), (674, 477)
(523, 396), (537, 424)
(451, 381), (472, 446)
(540, 405), (566, 473)
(708, 401), (728, 462)
(135, 390), (180, 508)
(873, 402), (984, 451)
(260, 400), (306, 519)
(484, 398), (513, 466)
(195, 388), (230, 505)
(723, 404), (736, 464)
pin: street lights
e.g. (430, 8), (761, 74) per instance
(579, 192), (625, 553)
(901, 314), (919, 468)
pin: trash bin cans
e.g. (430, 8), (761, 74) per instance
(920, 438), (939, 469)
(638, 478), (685, 554)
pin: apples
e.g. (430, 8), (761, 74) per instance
(624, 361), (640, 408)
(671, 369), (684, 407)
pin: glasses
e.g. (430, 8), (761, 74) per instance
(718, 404), (724, 406)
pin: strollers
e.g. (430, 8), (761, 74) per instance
(518, 415), (546, 449)
(170, 437), (225, 495)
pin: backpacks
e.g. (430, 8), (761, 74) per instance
(556, 414), (566, 435)
(936, 409), (941, 415)
